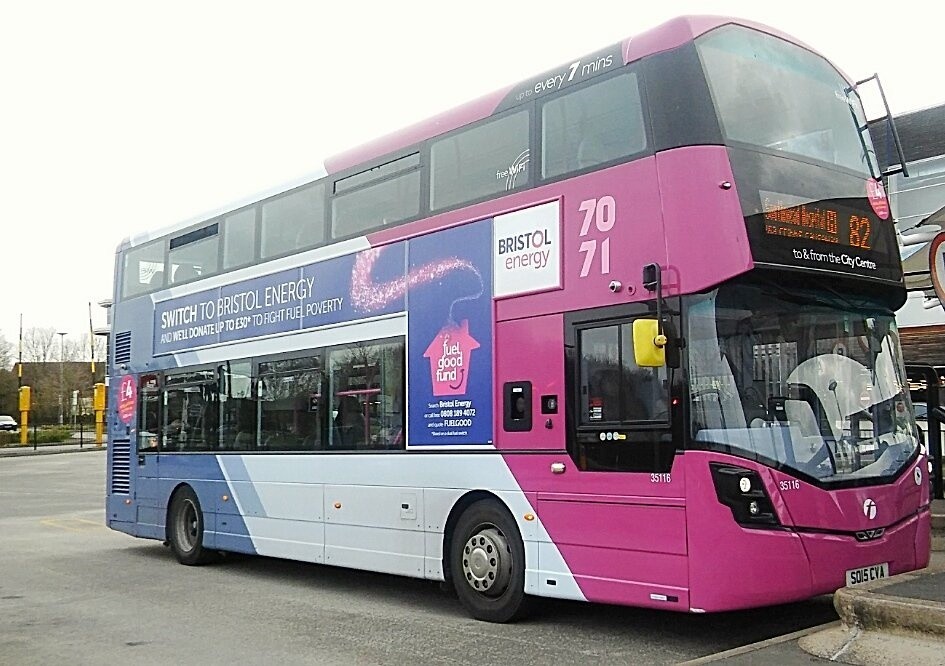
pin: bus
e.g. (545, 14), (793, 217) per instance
(105, 14), (931, 626)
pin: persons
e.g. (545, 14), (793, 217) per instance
(145, 392), (403, 447)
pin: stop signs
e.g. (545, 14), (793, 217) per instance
(860, 391), (870, 407)
(895, 400), (906, 417)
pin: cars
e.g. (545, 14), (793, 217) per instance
(0, 416), (18, 432)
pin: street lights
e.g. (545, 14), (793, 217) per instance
(828, 379), (844, 422)
(56, 332), (69, 426)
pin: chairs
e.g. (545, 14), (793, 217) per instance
(149, 264), (217, 288)
(218, 424), (364, 451)
